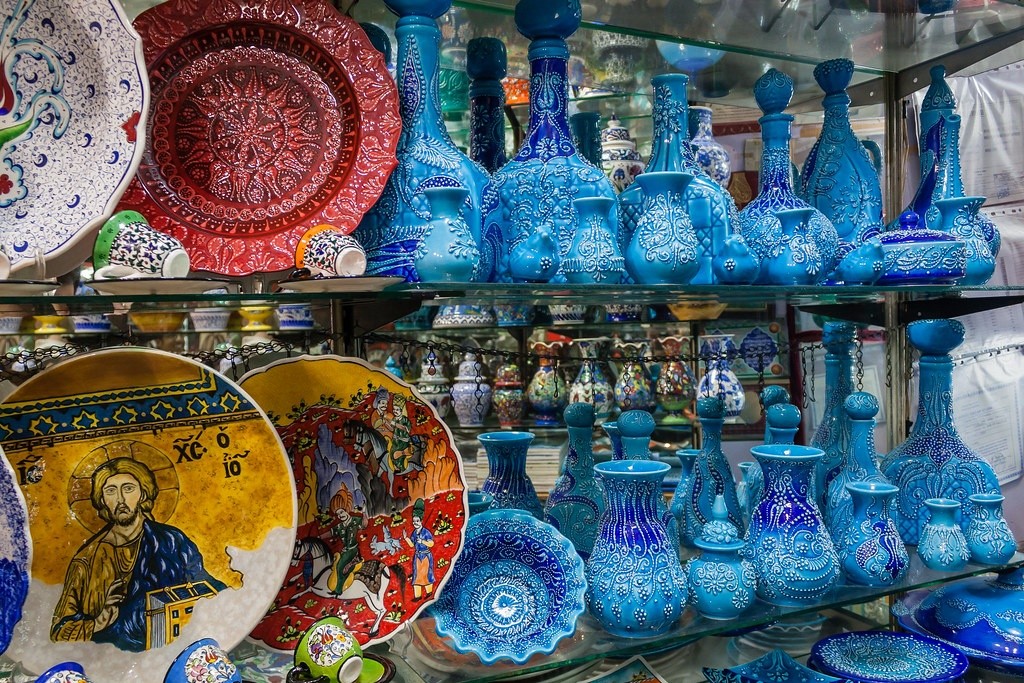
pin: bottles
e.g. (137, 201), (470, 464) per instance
(358, 0), (1002, 285)
(383, 333), (1018, 642)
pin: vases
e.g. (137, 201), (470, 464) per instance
(414, 187), (477, 284)
(935, 196), (995, 285)
(760, 208), (823, 285)
(464, 432), (1017, 639)
(688, 106), (731, 188)
(625, 172), (702, 285)
(562, 197), (624, 284)
(527, 333), (747, 425)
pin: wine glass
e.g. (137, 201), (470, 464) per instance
(238, 305), (273, 332)
(32, 314), (70, 333)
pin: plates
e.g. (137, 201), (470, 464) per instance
(1, 0), (151, 277)
(236, 352), (471, 659)
(426, 508), (589, 665)
(0, 279), (61, 298)
(116, 2), (402, 276)
(1, 343), (299, 683)
(277, 276), (406, 291)
(493, 615), (843, 683)
(890, 577), (1024, 668)
(83, 278), (232, 295)
(810, 628), (969, 683)
(354, 651), (398, 683)
(0, 444), (34, 660)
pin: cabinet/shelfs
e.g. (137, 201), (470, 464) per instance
(1, 0), (1024, 683)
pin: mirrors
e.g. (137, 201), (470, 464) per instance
(439, 68), (885, 214)
(348, 322), (898, 506)
(1, 303), (336, 406)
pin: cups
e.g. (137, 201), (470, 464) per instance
(293, 223), (367, 277)
(285, 615), (364, 683)
(92, 210), (192, 279)
(162, 637), (243, 683)
(35, 660), (94, 683)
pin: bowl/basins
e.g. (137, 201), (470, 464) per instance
(0, 300), (316, 336)
(392, 301), (728, 328)
